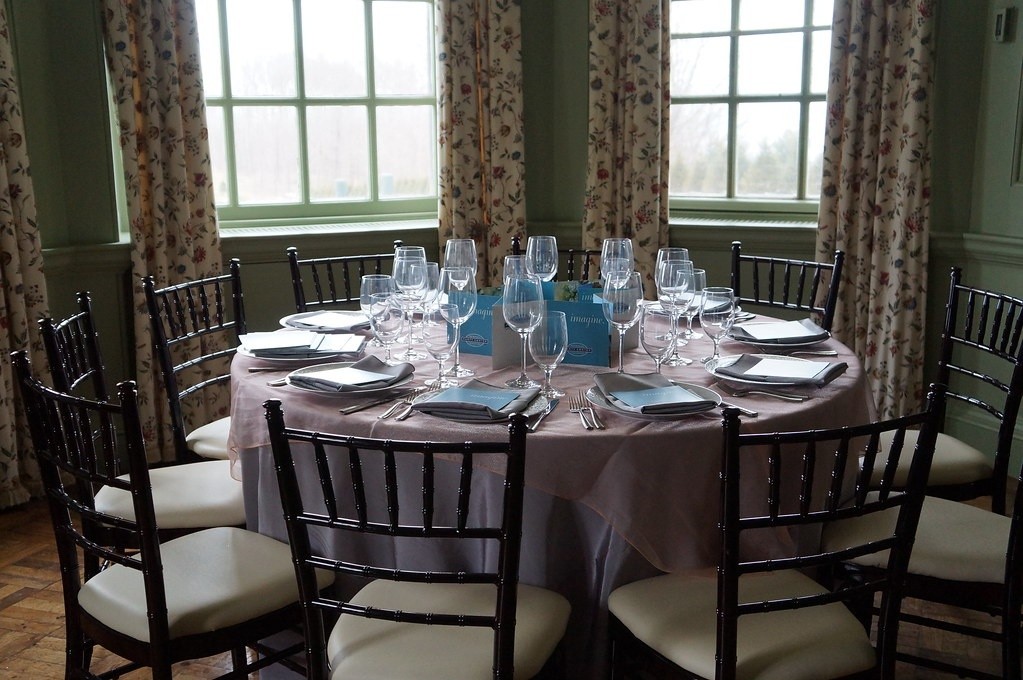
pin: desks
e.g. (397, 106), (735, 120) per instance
(225, 297), (881, 680)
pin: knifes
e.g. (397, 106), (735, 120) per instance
(527, 399), (560, 432)
(339, 386), (427, 414)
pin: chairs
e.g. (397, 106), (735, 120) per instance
(10, 235), (1022, 680)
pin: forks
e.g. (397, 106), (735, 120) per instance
(568, 390), (605, 430)
(378, 384), (441, 421)
(713, 378), (809, 401)
(752, 344), (837, 356)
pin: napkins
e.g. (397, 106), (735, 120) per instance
(285, 309), (372, 332)
(288, 355), (416, 393)
(720, 318), (829, 342)
(645, 296), (739, 316)
(593, 372), (718, 415)
(409, 378), (542, 420)
(714, 352), (849, 389)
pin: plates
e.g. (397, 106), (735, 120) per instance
(585, 383), (722, 422)
(650, 301), (741, 317)
(723, 323), (829, 346)
(413, 389), (548, 423)
(705, 354), (814, 384)
(235, 343), (340, 366)
(279, 310), (370, 332)
(286, 362), (414, 398)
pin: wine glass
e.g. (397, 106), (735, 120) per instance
(503, 235), (567, 399)
(654, 248), (706, 367)
(699, 287), (735, 364)
(601, 238), (644, 374)
(641, 303), (677, 382)
(360, 238), (477, 388)
